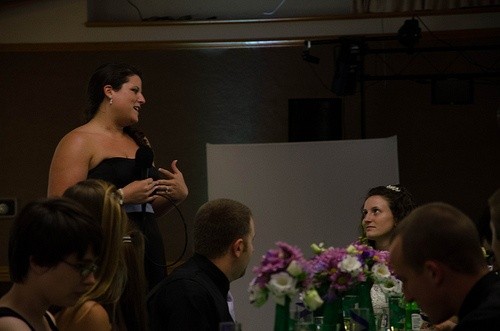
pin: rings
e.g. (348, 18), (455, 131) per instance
(166, 187), (170, 191)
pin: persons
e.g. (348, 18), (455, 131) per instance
(358, 182), (500, 331)
(0, 179), (256, 331)
(48, 62), (187, 331)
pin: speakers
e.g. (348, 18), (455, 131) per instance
(287, 97), (344, 142)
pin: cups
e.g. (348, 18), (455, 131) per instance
(219, 323), (241, 331)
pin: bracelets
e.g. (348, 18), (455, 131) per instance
(119, 188), (125, 204)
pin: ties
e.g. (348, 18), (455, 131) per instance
(227, 290), (235, 322)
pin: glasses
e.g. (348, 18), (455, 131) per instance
(61, 259), (104, 279)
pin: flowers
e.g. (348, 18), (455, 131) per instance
(249, 236), (402, 311)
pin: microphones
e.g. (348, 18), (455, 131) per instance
(135, 145), (153, 215)
